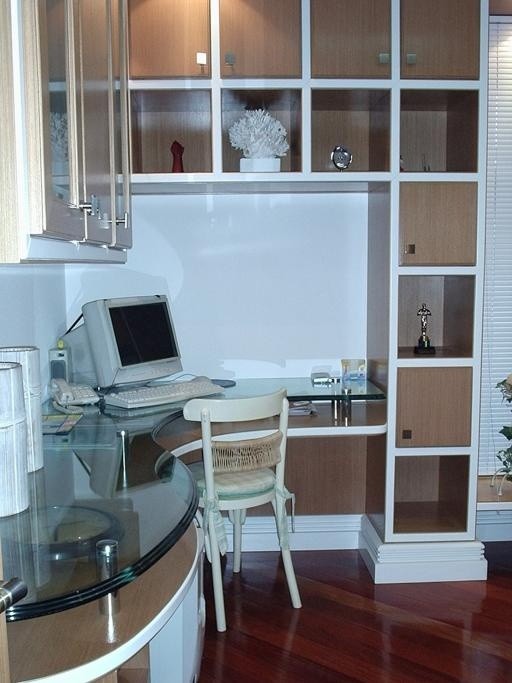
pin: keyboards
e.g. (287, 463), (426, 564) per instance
(104, 380), (225, 408)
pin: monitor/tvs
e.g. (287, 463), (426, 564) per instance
(57, 294), (184, 388)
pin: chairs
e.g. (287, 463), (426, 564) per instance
(183, 388), (303, 633)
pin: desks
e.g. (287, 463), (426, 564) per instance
(0, 375), (385, 682)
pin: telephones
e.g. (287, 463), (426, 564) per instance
(50, 378), (99, 406)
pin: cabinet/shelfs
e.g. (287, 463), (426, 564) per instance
(0, 0), (131, 262)
(50, 0), (489, 583)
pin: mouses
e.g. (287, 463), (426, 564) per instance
(192, 375), (211, 384)
